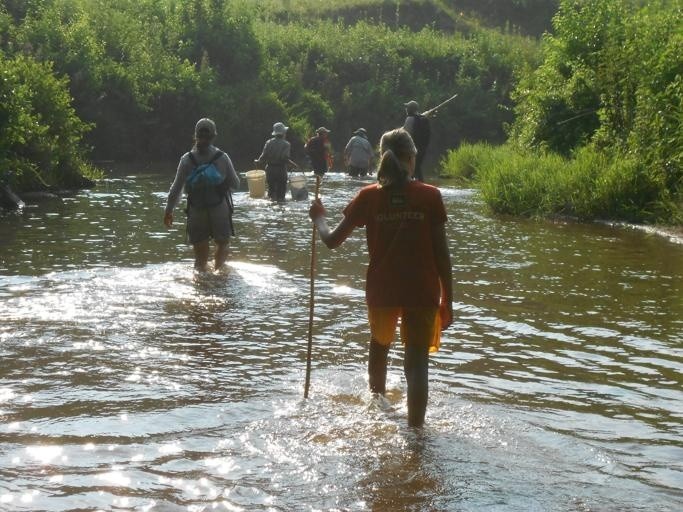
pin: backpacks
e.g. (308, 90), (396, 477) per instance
(184, 150), (225, 207)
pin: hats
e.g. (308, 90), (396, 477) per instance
(353, 128), (368, 139)
(316, 127), (330, 134)
(195, 118), (217, 137)
(402, 101), (419, 109)
(272, 122), (289, 136)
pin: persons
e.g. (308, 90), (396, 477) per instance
(304, 127), (331, 178)
(164, 118), (240, 271)
(254, 123), (296, 201)
(403, 100), (426, 182)
(309, 130), (453, 429)
(344, 127), (373, 177)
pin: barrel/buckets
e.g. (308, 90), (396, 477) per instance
(289, 175), (308, 200)
(246, 170), (266, 198)
